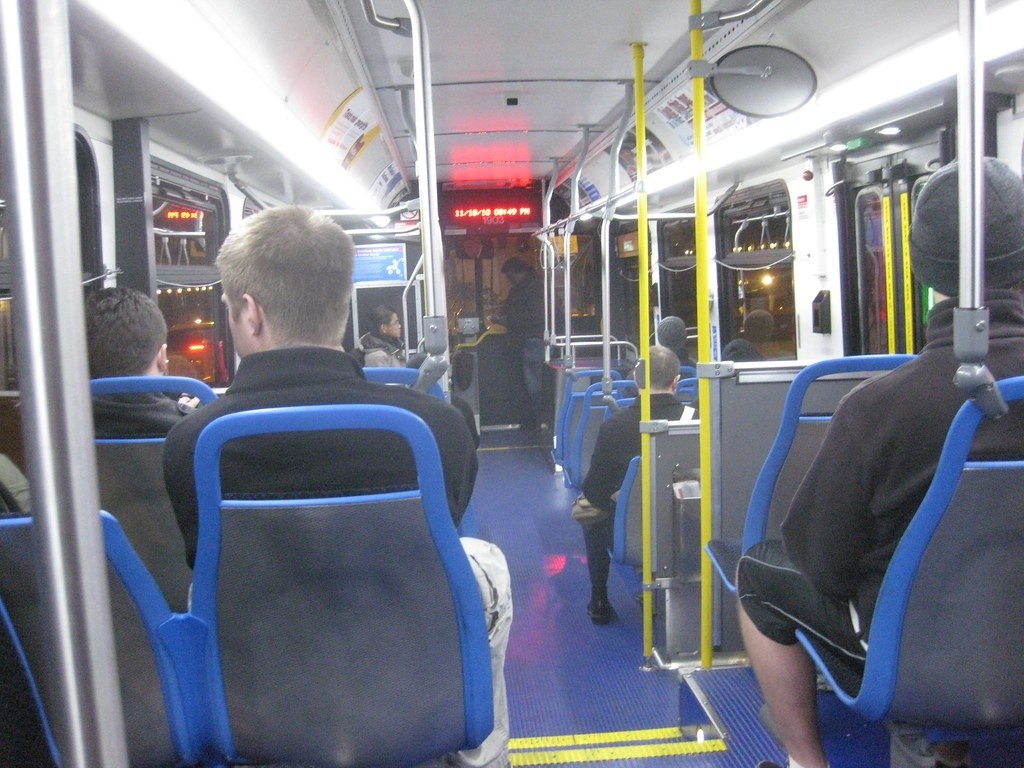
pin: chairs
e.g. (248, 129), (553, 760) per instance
(556, 354), (1024, 768)
(0, 365), (503, 768)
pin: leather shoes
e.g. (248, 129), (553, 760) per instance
(584, 597), (611, 623)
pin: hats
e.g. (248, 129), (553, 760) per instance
(909, 155), (1023, 295)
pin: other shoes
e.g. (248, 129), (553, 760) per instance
(931, 739), (971, 766)
(755, 761), (782, 768)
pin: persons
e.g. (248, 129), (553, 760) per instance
(737, 158), (1024, 767)
(358, 306), (480, 449)
(87, 285), (193, 438)
(658, 316), (697, 367)
(722, 339), (769, 360)
(164, 206), (513, 767)
(491, 256), (551, 430)
(581, 347), (700, 624)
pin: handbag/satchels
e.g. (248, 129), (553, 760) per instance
(571, 489), (620, 525)
(521, 344), (550, 397)
